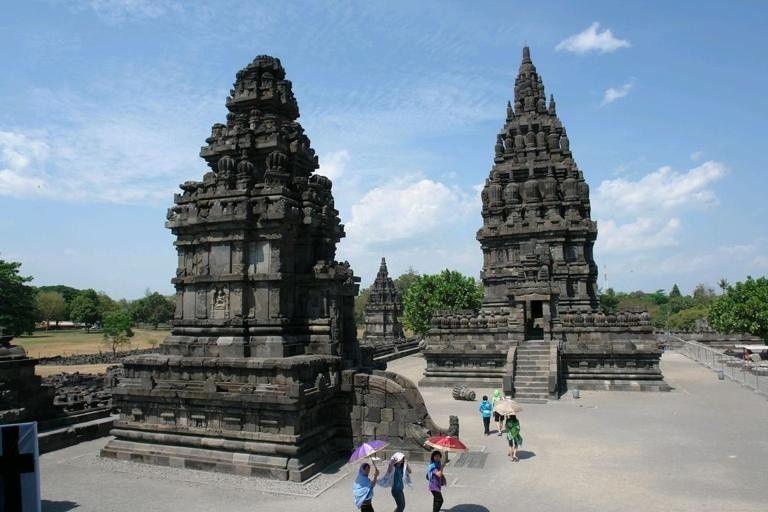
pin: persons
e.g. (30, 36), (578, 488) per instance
(388, 452), (412, 512)
(492, 390), (506, 435)
(743, 350), (750, 361)
(355, 463), (379, 511)
(505, 415), (520, 460)
(427, 450), (448, 511)
(479, 394), (492, 435)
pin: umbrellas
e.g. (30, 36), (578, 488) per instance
(349, 439), (390, 470)
(425, 435), (470, 460)
(494, 398), (522, 418)
